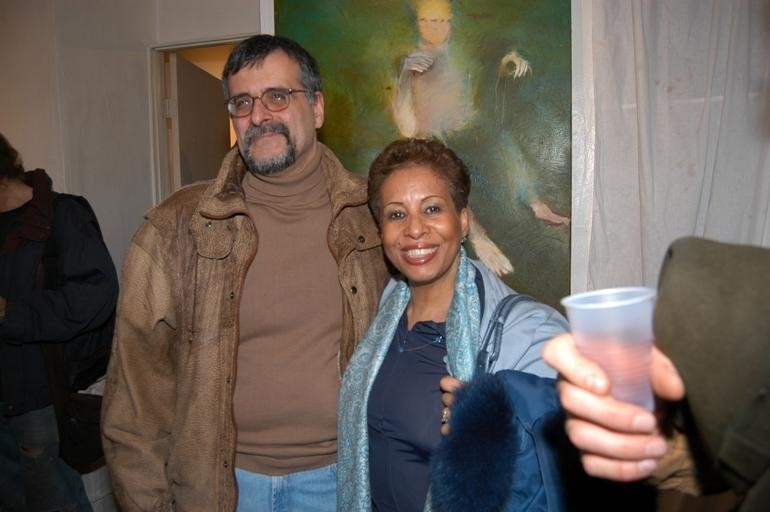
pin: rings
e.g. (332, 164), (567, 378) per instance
(441, 411), (448, 423)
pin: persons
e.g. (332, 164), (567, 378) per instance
(540, 234), (748, 510)
(0, 131), (120, 512)
(99, 56), (391, 511)
(383, 1), (569, 277)
(337, 137), (572, 512)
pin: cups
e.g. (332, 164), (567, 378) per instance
(559, 286), (660, 417)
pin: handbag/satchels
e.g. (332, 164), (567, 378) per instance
(58, 391), (103, 473)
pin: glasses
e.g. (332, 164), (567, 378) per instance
(225, 87), (308, 118)
